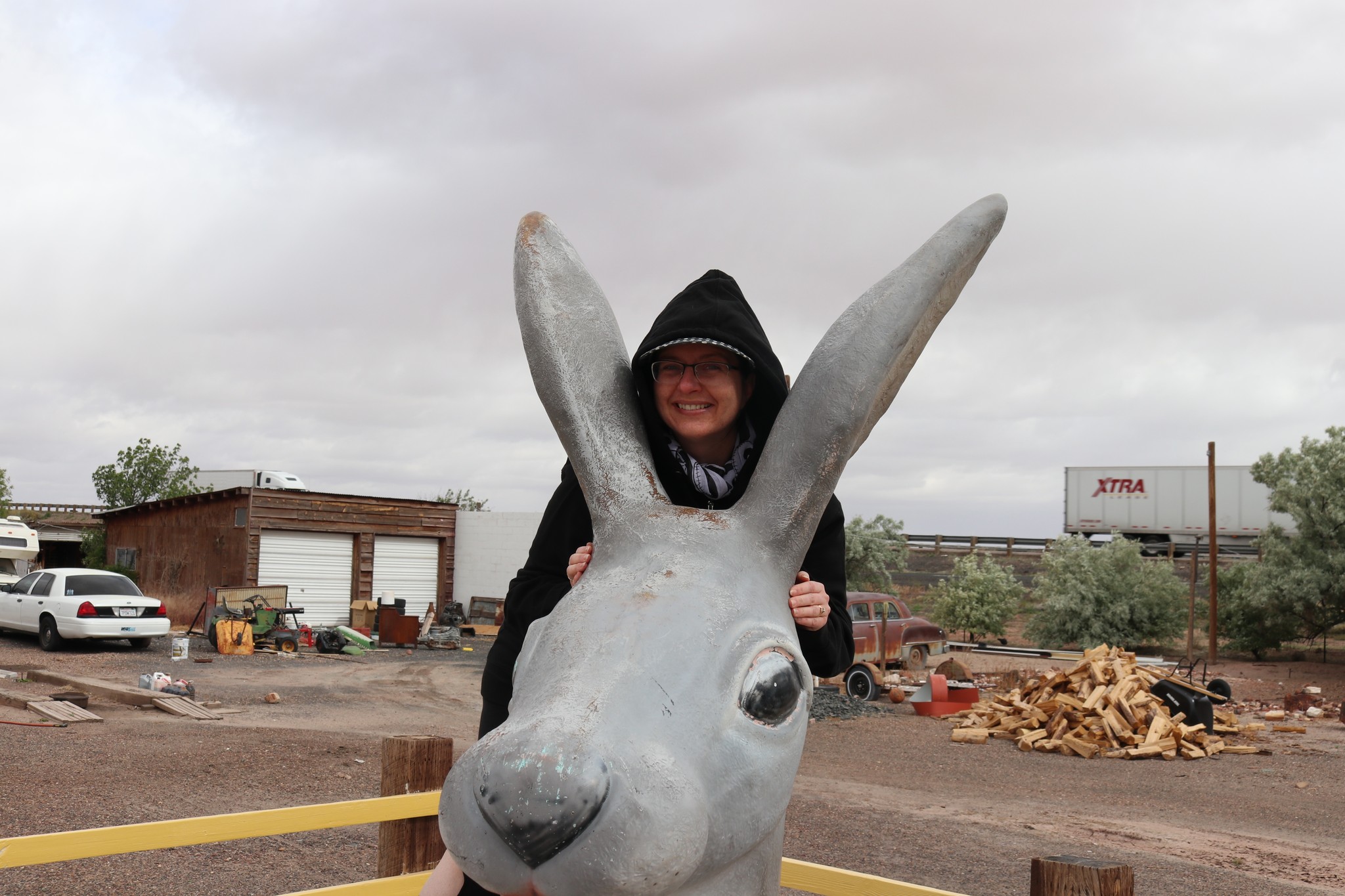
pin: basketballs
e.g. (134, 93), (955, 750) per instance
(889, 687), (905, 703)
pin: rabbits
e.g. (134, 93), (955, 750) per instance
(429, 189), (1010, 896)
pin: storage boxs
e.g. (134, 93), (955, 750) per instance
(350, 600), (378, 631)
(352, 627), (371, 639)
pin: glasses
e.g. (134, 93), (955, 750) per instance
(650, 359), (738, 385)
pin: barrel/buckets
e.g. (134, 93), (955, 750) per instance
(171, 631), (190, 659)
(138, 673), (153, 689)
(495, 599), (505, 625)
(151, 672), (171, 691)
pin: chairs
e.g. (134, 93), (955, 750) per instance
(379, 607), (419, 649)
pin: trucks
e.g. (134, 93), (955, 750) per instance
(160, 469), (308, 500)
(1061, 463), (1316, 558)
(0, 515), (40, 588)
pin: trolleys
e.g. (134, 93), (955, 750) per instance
(1137, 655), (1233, 746)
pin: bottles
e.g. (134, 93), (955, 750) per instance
(155, 677), (195, 701)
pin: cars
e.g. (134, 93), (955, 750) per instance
(0, 566), (170, 652)
(845, 591), (951, 671)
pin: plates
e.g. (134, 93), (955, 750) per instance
(194, 658), (213, 662)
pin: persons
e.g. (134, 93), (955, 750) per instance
(453, 267), (856, 896)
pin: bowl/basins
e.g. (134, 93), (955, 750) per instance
(49, 692), (89, 708)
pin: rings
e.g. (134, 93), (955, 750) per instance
(818, 605), (826, 617)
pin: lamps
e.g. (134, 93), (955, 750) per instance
(381, 591), (395, 607)
